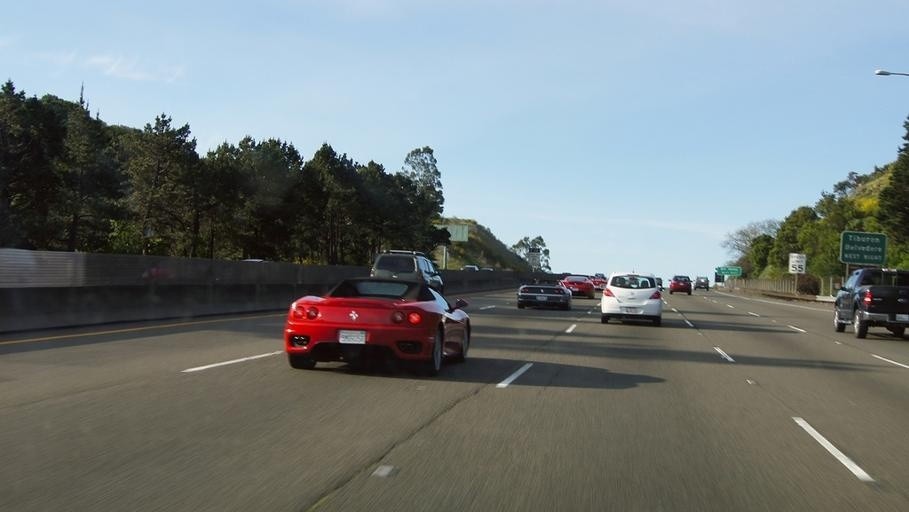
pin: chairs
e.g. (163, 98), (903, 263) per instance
(641, 281), (648, 288)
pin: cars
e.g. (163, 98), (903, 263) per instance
(460, 265), (513, 272)
(516, 272), (664, 325)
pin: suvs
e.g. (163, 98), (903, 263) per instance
(372, 249), (445, 294)
(669, 275), (693, 295)
(693, 276), (710, 291)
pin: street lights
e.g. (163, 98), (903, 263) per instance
(875, 69), (909, 78)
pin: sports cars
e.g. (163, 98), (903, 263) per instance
(281, 274), (472, 376)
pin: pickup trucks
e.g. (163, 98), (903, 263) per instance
(833, 266), (909, 340)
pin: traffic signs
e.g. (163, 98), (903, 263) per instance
(788, 253), (806, 274)
(716, 266), (742, 277)
(839, 230), (887, 266)
